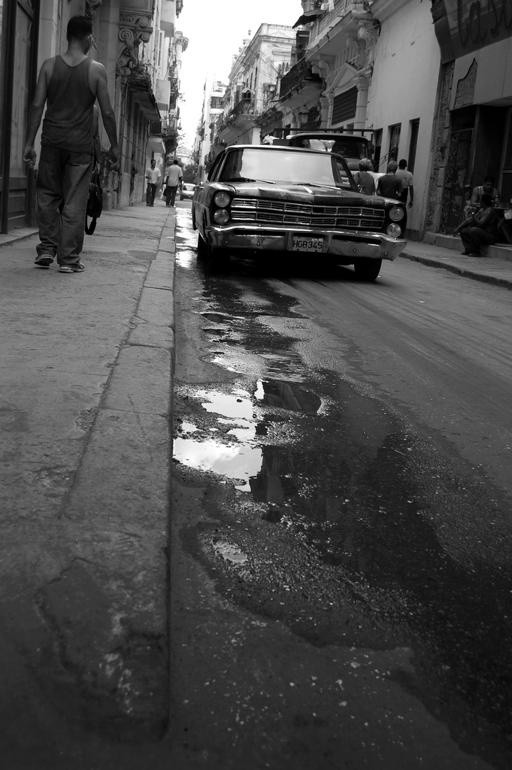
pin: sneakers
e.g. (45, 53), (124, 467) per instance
(59, 262), (85, 272)
(35, 254), (55, 266)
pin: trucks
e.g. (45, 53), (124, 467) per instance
(261, 127), (395, 195)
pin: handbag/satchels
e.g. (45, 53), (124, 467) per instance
(87, 181), (102, 218)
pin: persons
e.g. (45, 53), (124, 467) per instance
(166, 160), (183, 207)
(470, 179), (499, 208)
(354, 158), (376, 196)
(145, 159), (161, 207)
(377, 160), (403, 199)
(454, 197), (498, 257)
(395, 159), (414, 209)
(24, 15), (119, 272)
(54, 103), (100, 263)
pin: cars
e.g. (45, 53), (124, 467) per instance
(191, 144), (409, 282)
(179, 182), (196, 201)
